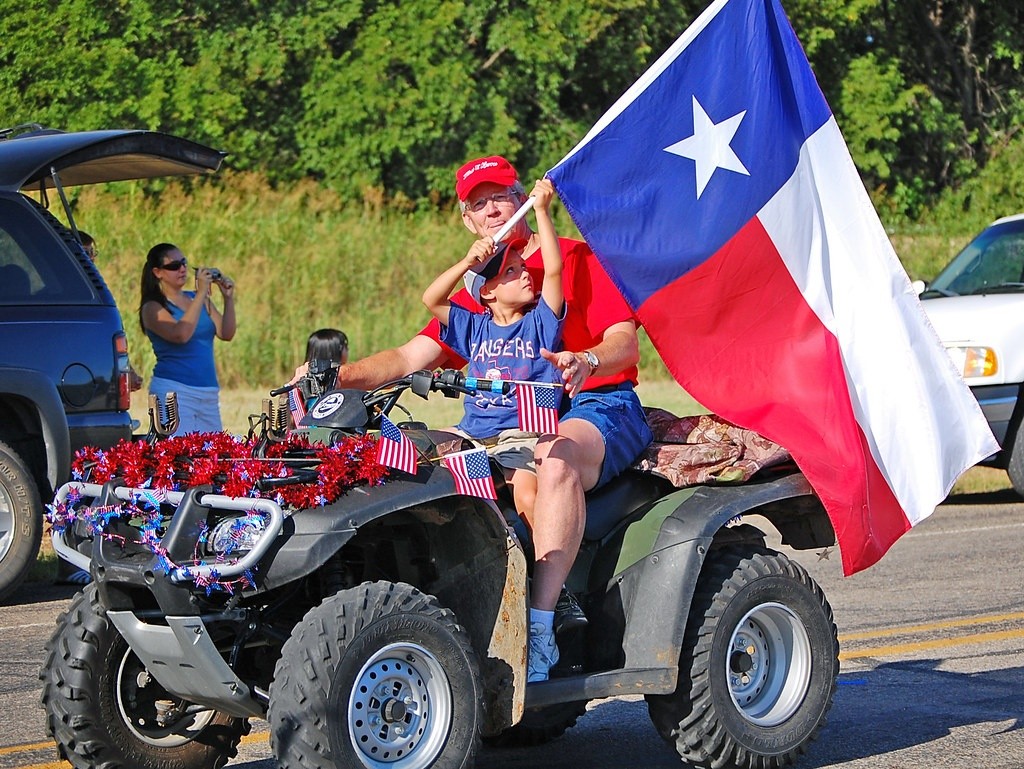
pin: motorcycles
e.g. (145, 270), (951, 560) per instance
(38, 359), (867, 769)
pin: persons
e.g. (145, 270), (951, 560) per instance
(305, 328), (350, 368)
(36, 229), (142, 394)
(138, 243), (237, 438)
(421, 180), (586, 636)
(284, 156), (650, 685)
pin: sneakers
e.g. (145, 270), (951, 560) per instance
(526, 621), (559, 684)
(553, 587), (588, 632)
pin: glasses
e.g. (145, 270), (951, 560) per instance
(84, 250), (99, 257)
(156, 257), (187, 272)
(463, 189), (520, 212)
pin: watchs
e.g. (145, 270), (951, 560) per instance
(582, 349), (599, 379)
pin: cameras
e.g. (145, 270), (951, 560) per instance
(206, 268), (220, 280)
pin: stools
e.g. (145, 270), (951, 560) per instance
(563, 467), (666, 595)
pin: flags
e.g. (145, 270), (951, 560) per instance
(377, 410), (417, 475)
(443, 448), (498, 500)
(289, 389), (310, 429)
(543, 0), (1003, 576)
(514, 382), (559, 435)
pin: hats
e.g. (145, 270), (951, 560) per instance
(455, 156), (519, 202)
(463, 238), (521, 306)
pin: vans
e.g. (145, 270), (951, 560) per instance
(0, 121), (231, 612)
(908, 212), (1023, 504)
